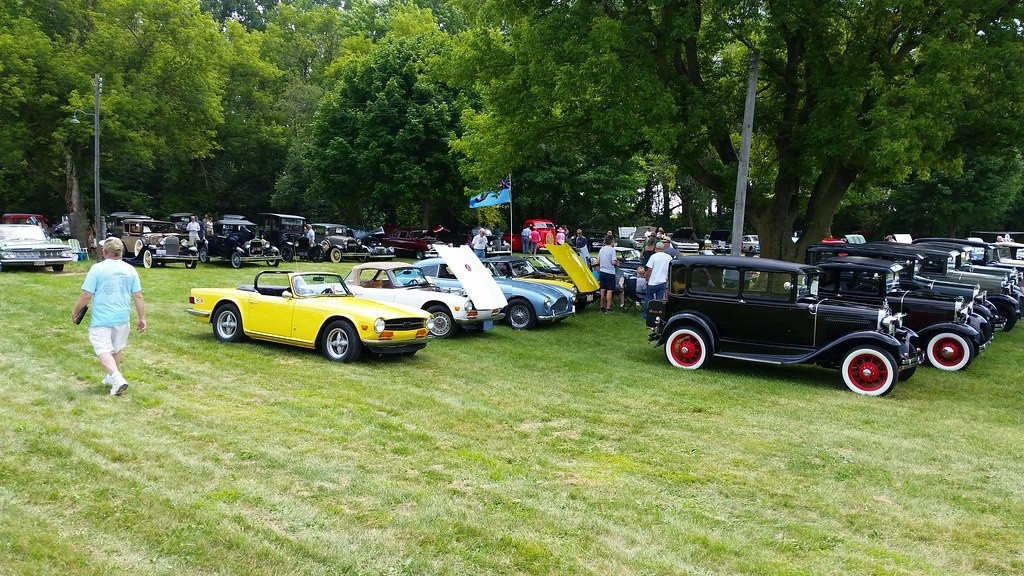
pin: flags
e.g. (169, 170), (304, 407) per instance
(469, 174), (511, 208)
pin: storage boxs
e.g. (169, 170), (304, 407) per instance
(77, 252), (87, 260)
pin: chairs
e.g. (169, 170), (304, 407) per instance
(51, 238), (62, 244)
(68, 238), (90, 260)
(592, 290), (618, 308)
(623, 276), (645, 314)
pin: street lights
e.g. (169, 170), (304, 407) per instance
(71, 74), (107, 262)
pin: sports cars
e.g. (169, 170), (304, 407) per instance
(381, 256), (579, 330)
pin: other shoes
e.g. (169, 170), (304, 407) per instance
(646, 325), (657, 330)
(620, 303), (624, 307)
(605, 308), (614, 315)
(636, 301), (640, 307)
(600, 306), (606, 312)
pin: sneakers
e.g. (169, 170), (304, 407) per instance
(102, 374), (114, 385)
(111, 377), (128, 396)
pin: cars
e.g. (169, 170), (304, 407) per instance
(297, 244), (509, 340)
(0, 222), (72, 272)
(187, 270), (435, 363)
(2, 214), (60, 239)
(308, 223), (369, 263)
(118, 218), (200, 270)
(54, 221), (72, 240)
(795, 230), (1024, 374)
(197, 219), (282, 268)
(463, 218), (760, 308)
(346, 224), (397, 262)
(383, 223), (452, 260)
(256, 211), (325, 263)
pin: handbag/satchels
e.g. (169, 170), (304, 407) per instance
(76, 306), (88, 325)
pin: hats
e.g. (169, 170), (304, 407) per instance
(662, 236), (671, 242)
(658, 227), (663, 231)
(99, 237), (123, 256)
(557, 227), (565, 232)
(607, 231), (612, 235)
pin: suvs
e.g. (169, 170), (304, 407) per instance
(646, 256), (927, 401)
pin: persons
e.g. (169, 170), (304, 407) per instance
(495, 226), (501, 237)
(522, 223), (683, 329)
(71, 237), (147, 397)
(26, 215), (71, 237)
(297, 224), (315, 261)
(885, 235), (897, 243)
(472, 228), (488, 262)
(995, 232), (1010, 243)
(186, 215), (213, 244)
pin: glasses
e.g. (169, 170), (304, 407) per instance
(577, 233), (581, 234)
